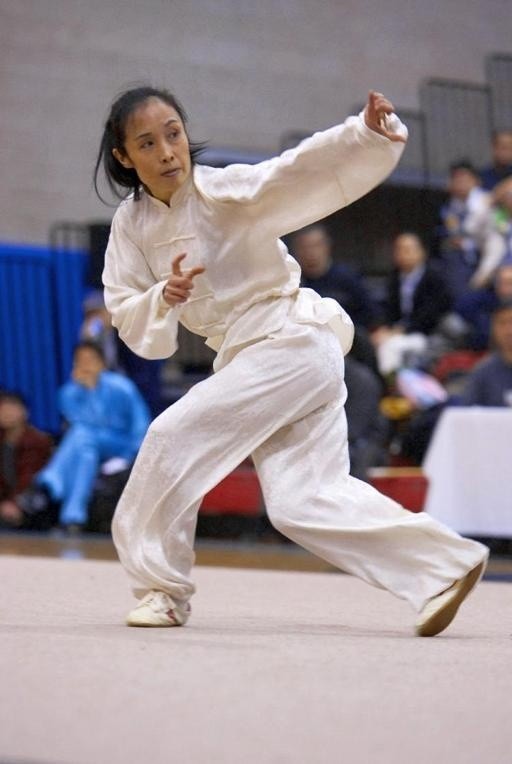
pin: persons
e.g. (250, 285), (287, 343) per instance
(92, 85), (491, 635)
(0, 392), (55, 500)
(0, 341), (150, 538)
(79, 292), (127, 370)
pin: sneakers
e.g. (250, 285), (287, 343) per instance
(127, 592), (196, 629)
(416, 550), (487, 638)
